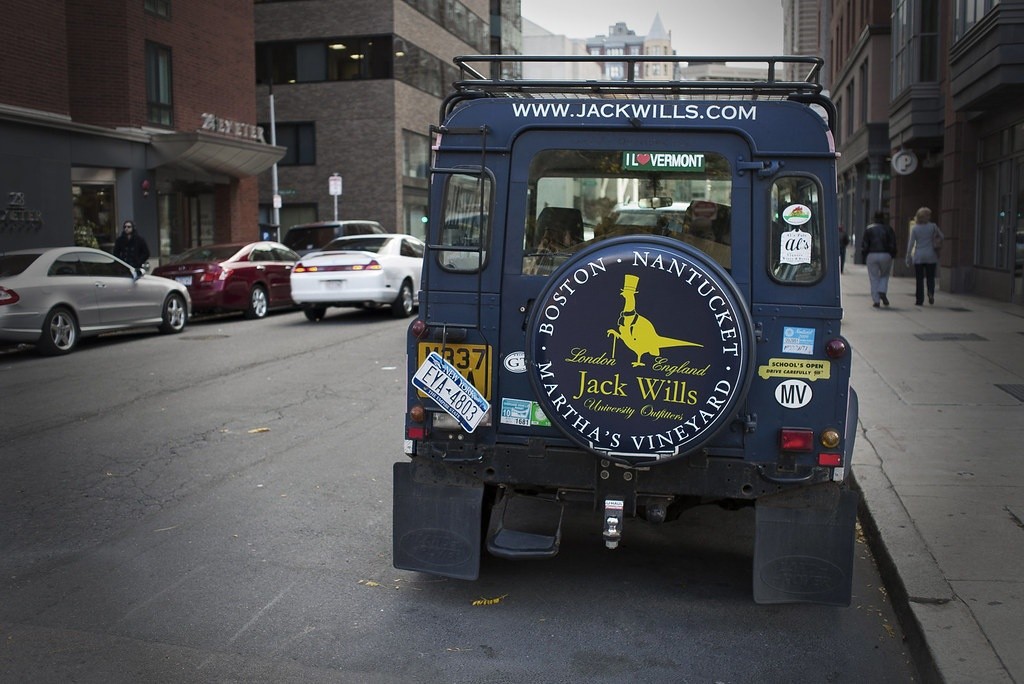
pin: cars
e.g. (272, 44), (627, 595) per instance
(149, 241), (307, 321)
(0, 247), (192, 355)
(288, 233), (426, 321)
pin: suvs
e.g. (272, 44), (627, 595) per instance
(392, 53), (862, 608)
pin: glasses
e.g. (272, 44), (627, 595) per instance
(124, 226), (132, 228)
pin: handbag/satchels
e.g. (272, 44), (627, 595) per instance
(933, 223), (942, 251)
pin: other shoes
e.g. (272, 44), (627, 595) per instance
(880, 294), (889, 306)
(916, 299), (923, 305)
(872, 303), (881, 307)
(930, 296), (935, 304)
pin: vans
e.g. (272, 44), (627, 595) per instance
(282, 220), (386, 263)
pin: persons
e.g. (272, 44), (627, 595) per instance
(838, 223), (850, 275)
(113, 220), (151, 270)
(905, 207), (945, 306)
(861, 210), (898, 308)
